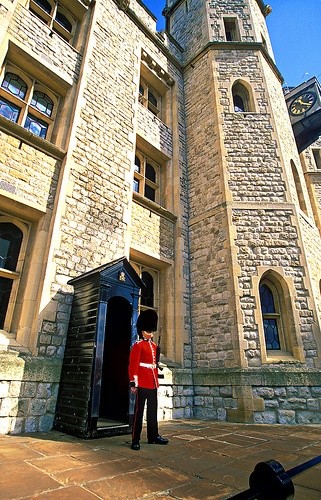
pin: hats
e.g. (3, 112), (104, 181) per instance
(136, 309), (158, 341)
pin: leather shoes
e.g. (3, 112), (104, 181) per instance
(147, 436), (168, 445)
(131, 440), (140, 450)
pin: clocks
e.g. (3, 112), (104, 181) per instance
(288, 91), (317, 116)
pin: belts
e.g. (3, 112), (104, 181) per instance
(138, 363), (156, 370)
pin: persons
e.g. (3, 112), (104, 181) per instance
(127, 309), (170, 450)
